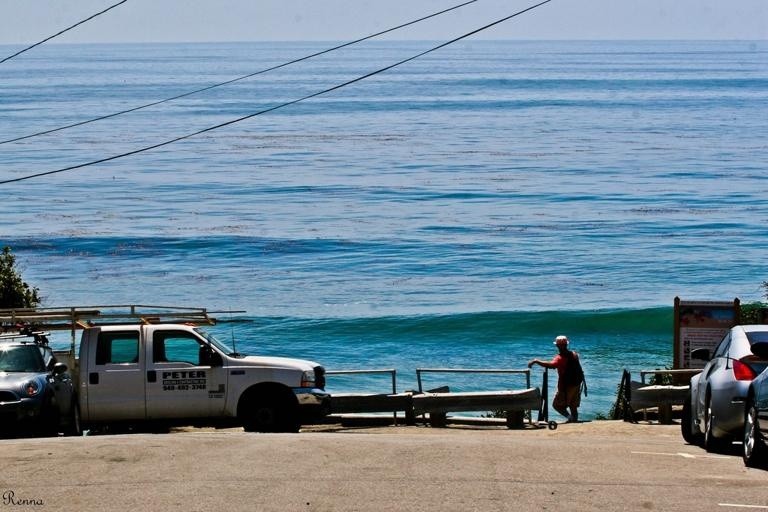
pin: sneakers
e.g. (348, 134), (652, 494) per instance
(565, 418), (575, 423)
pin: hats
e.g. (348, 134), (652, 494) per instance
(553, 335), (569, 345)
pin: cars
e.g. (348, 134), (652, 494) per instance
(1, 339), (80, 439)
(742, 342), (767, 469)
(681, 325), (767, 453)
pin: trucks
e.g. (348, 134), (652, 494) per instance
(1, 302), (333, 434)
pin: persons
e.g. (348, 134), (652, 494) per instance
(528, 335), (584, 423)
(45, 362), (77, 437)
